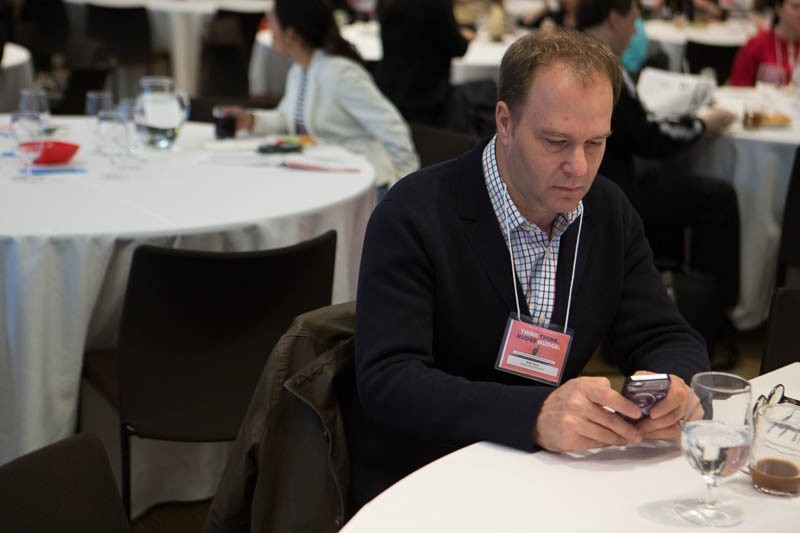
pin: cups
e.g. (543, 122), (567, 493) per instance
(751, 404), (800, 497)
(213, 106), (239, 141)
(743, 92), (767, 132)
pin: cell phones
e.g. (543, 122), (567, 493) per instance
(614, 374), (670, 425)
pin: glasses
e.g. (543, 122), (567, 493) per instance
(753, 384), (800, 434)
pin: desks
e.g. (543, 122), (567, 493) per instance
(0, 113), (376, 511)
(641, 78), (800, 328)
(340, 362), (800, 533)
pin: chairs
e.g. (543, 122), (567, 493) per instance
(0, 0), (686, 533)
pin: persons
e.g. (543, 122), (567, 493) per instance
(728, 0), (800, 90)
(376, 0), (478, 132)
(211, 0), (419, 187)
(351, 29), (712, 509)
(571, 1), (742, 372)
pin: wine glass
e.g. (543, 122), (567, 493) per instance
(8, 76), (190, 185)
(678, 372), (753, 527)
